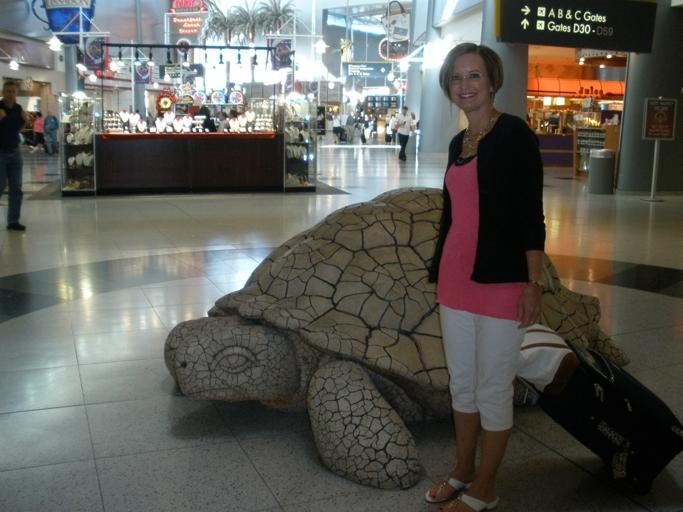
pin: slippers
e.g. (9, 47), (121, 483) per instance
(425, 476), (471, 503)
(438, 492), (499, 512)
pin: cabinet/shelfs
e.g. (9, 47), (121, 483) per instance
(279, 102), (317, 192)
(58, 97), (97, 196)
(572, 124), (620, 179)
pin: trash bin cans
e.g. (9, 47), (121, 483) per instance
(587, 148), (615, 194)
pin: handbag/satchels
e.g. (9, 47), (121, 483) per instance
(514, 324), (579, 394)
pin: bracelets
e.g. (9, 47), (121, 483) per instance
(527, 280), (546, 295)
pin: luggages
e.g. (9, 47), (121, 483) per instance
(536, 338), (682, 495)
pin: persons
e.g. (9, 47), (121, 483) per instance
(118, 104), (255, 133)
(0, 81), (32, 231)
(425, 43), (546, 511)
(526, 107), (575, 134)
(20, 111), (59, 154)
(327, 106), (421, 161)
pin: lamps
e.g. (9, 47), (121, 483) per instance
(105, 43), (276, 65)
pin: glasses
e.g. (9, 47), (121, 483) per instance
(446, 71), (488, 82)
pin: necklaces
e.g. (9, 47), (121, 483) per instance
(462, 107), (497, 154)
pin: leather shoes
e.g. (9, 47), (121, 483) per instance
(7, 223), (26, 230)
